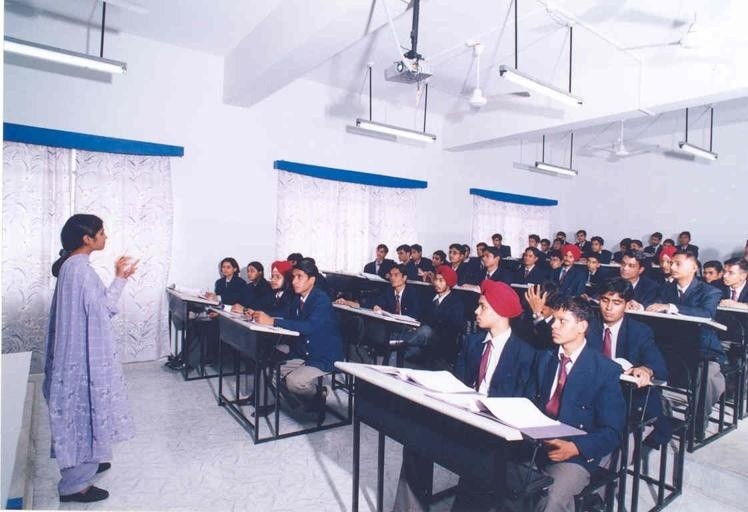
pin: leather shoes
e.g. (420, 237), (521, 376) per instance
(96, 463), (110, 473)
(250, 406), (275, 417)
(244, 394), (254, 405)
(165, 351), (193, 370)
(60, 486), (108, 503)
(387, 338), (407, 350)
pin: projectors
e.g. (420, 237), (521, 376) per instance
(384, 58), (433, 84)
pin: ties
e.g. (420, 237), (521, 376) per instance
(602, 328), (610, 358)
(479, 261), (566, 286)
(394, 295), (399, 315)
(475, 340), (491, 392)
(731, 290), (735, 299)
(298, 301), (304, 313)
(433, 298), (438, 304)
(544, 353), (570, 416)
(678, 289), (683, 303)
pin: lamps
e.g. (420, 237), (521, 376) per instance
(534, 133), (581, 178)
(677, 107), (720, 161)
(4, 1), (129, 74)
(353, 61), (438, 143)
(499, 1), (584, 109)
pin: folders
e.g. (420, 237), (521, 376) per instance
(425, 393), (588, 439)
(366, 365), (481, 394)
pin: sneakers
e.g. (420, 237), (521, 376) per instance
(303, 399), (312, 412)
(312, 385), (330, 412)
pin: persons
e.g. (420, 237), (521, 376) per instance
(166, 252), (345, 417)
(336, 229), (748, 512)
(40, 214), (139, 502)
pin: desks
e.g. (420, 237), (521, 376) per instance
(165, 286), (219, 382)
(321, 252), (736, 512)
(210, 307), (301, 445)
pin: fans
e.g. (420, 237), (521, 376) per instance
(590, 118), (659, 158)
(433, 43), (532, 107)
